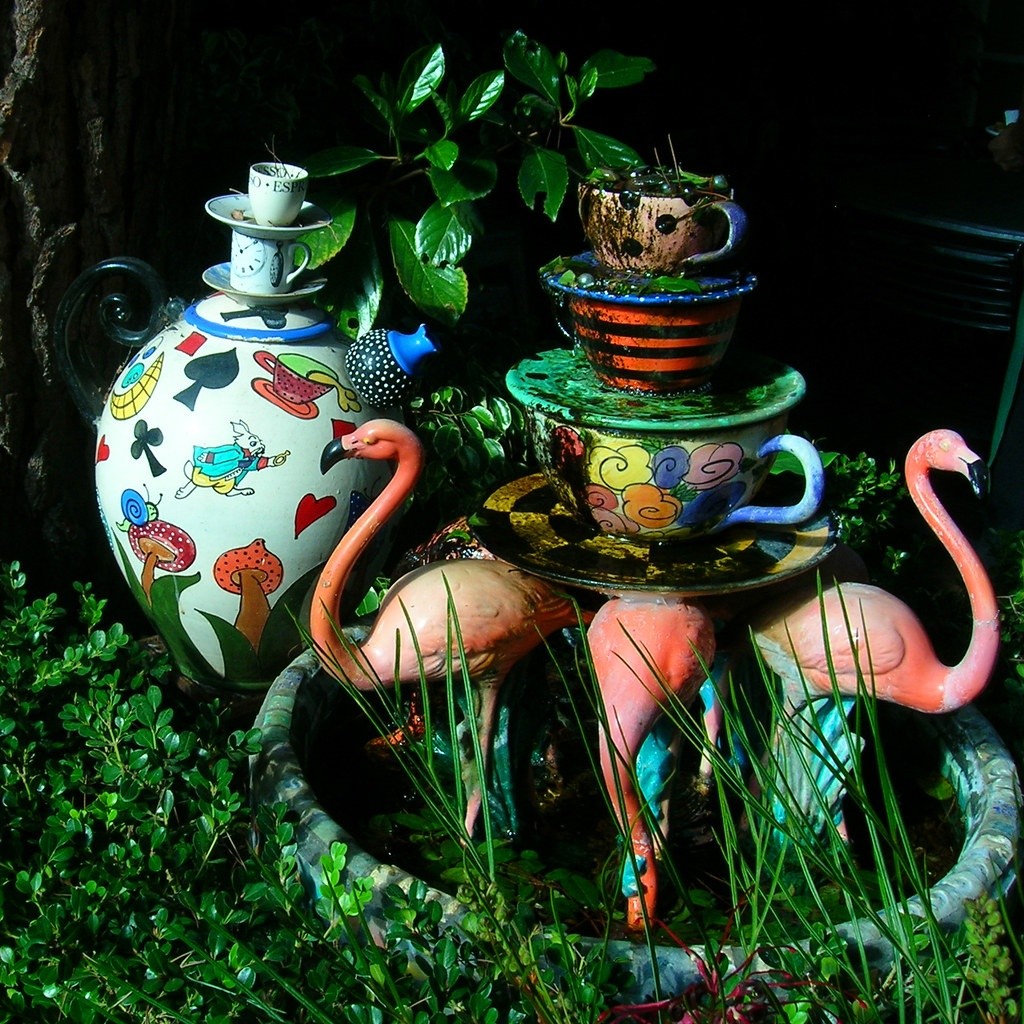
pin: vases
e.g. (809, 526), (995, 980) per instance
(43, 255), (414, 711)
(243, 623), (1022, 1010)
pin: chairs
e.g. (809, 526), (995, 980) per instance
(840, 203), (1024, 471)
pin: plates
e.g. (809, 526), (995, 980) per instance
(202, 262), (328, 308)
(204, 194), (334, 240)
(504, 347), (806, 431)
(472, 471), (843, 596)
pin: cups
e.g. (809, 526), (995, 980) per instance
(230, 230), (312, 294)
(506, 411), (824, 542)
(569, 300), (742, 395)
(544, 252), (760, 308)
(248, 163), (310, 227)
(580, 173), (747, 273)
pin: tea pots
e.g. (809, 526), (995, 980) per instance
(52, 255), (440, 695)
